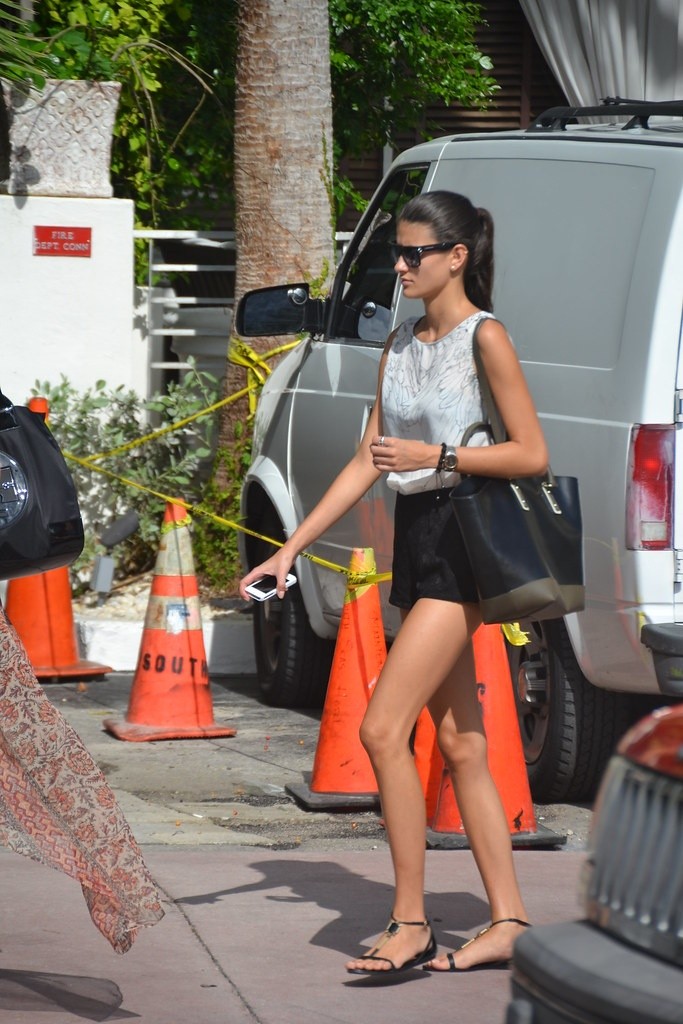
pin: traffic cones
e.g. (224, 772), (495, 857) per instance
(6, 396), (116, 682)
(410, 619), (566, 849)
(101, 497), (236, 745)
(286, 545), (392, 811)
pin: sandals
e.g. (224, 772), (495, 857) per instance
(348, 911), (438, 976)
(423, 918), (533, 971)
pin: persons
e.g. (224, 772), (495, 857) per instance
(239, 190), (551, 974)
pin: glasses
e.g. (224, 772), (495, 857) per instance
(385, 238), (459, 268)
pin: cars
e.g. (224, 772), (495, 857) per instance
(503, 694), (683, 1023)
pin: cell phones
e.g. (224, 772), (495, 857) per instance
(245, 573), (297, 602)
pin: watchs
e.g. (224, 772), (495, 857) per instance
(444, 446), (459, 474)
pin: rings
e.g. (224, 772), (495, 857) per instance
(379, 436), (385, 446)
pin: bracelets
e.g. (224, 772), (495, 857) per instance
(436, 442), (448, 473)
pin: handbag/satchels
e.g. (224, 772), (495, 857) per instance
(445, 422), (587, 625)
(0, 387), (87, 582)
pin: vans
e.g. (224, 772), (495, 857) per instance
(230, 100), (683, 803)
